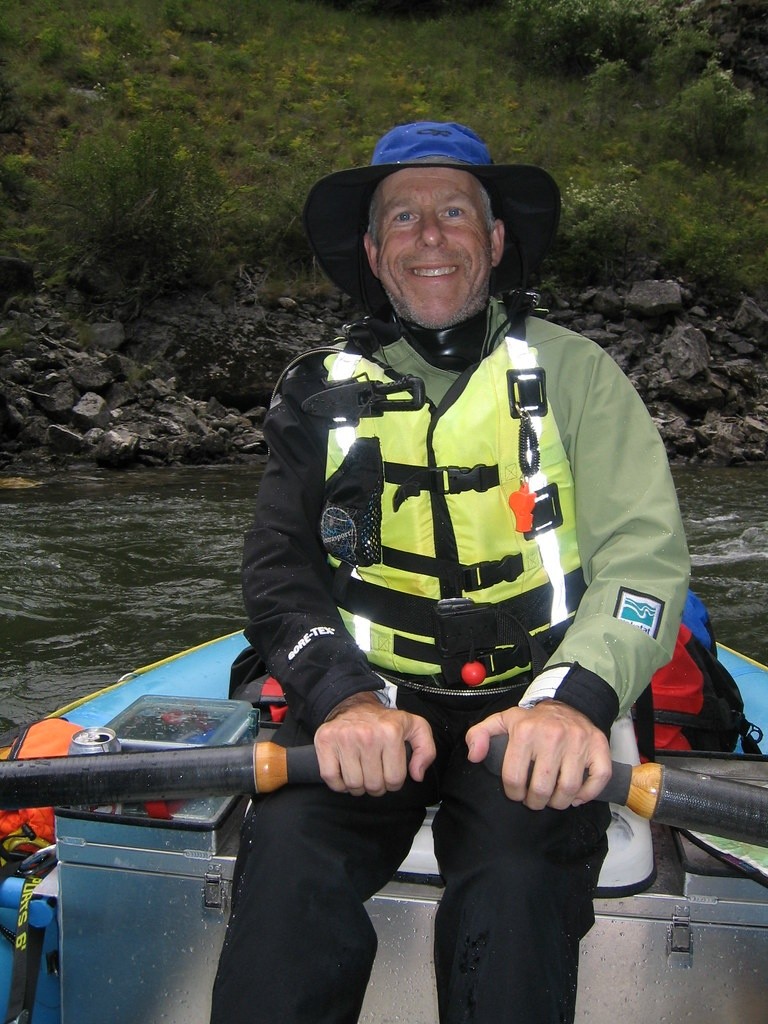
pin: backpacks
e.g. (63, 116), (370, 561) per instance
(631, 622), (763, 768)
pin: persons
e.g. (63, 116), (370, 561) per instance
(209, 121), (691, 1024)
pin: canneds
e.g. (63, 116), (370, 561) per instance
(69, 726), (123, 817)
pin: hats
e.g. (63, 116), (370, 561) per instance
(305, 121), (561, 321)
